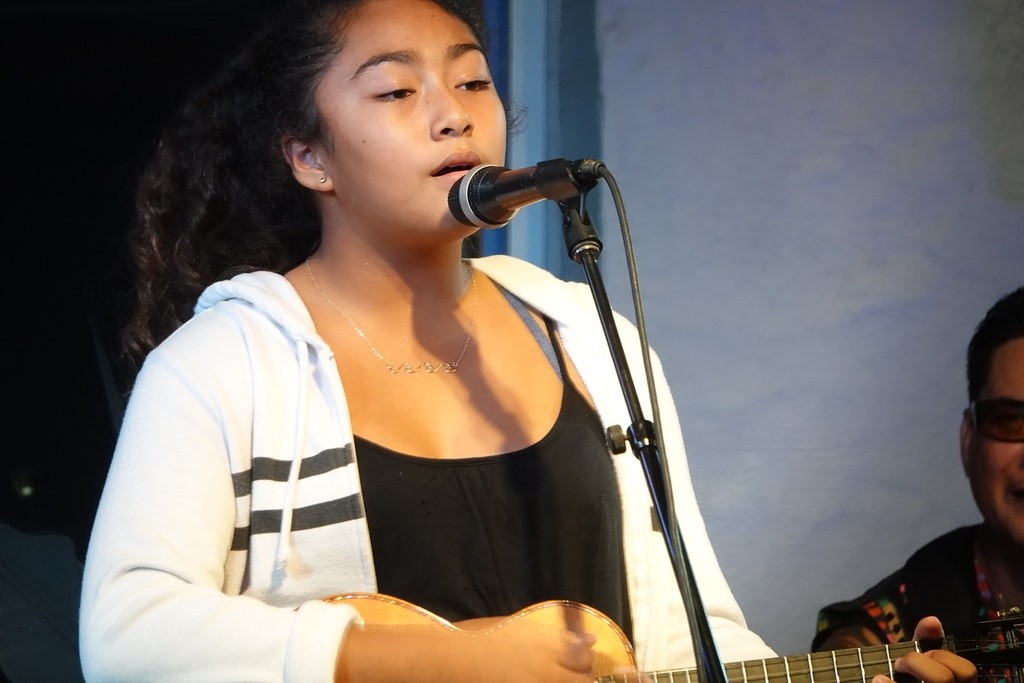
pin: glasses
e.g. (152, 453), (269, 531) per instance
(964, 397), (1024, 443)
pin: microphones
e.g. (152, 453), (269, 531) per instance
(448, 159), (603, 230)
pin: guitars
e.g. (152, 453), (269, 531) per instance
(291, 594), (1024, 683)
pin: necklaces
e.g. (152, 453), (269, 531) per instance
(301, 246), (475, 375)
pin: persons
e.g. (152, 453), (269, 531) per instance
(79, 0), (977, 683)
(805, 287), (1024, 682)
